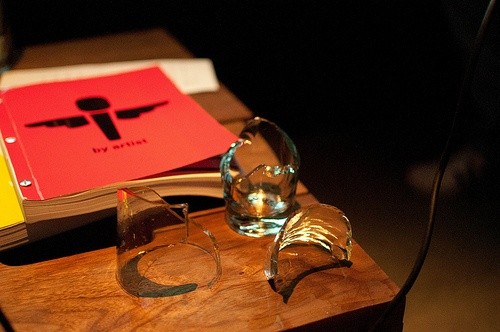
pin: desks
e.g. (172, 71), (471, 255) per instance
(0, 29), (406, 332)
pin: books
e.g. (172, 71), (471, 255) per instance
(0, 139), (115, 252)
(0, 66), (239, 224)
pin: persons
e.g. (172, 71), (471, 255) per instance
(410, 0), (500, 198)
